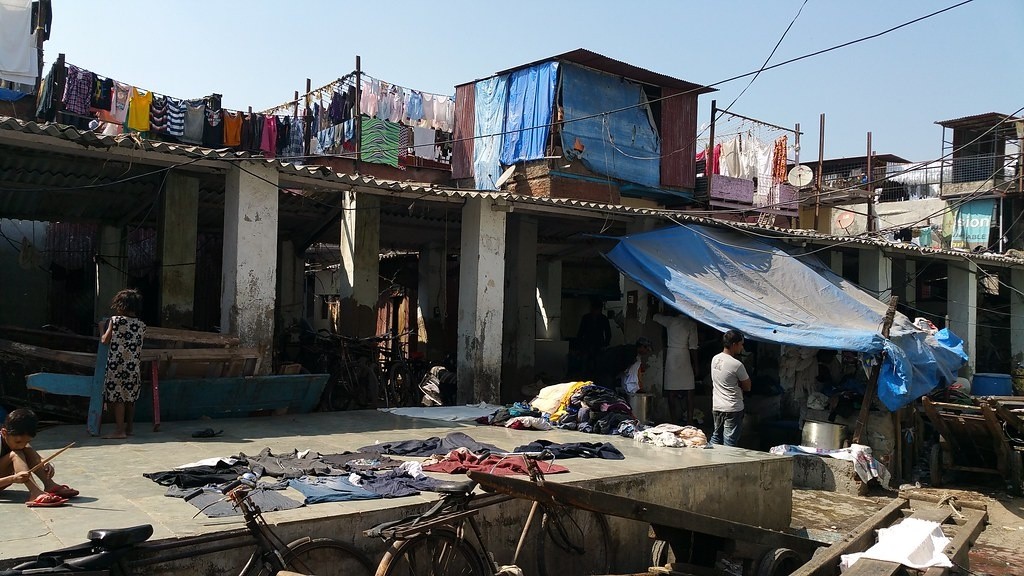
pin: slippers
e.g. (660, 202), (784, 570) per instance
(25, 493), (70, 506)
(47, 485), (80, 495)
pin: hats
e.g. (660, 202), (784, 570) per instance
(638, 337), (652, 352)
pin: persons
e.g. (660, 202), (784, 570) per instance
(648, 294), (701, 428)
(704, 328), (752, 448)
(574, 299), (612, 382)
(97, 287), (147, 440)
(0, 406), (80, 508)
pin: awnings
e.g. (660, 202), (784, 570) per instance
(601, 220), (970, 447)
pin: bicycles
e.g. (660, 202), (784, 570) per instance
(0, 470), (375, 576)
(285, 324), (422, 412)
(364, 451), (614, 576)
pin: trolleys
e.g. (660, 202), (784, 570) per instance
(921, 395), (1024, 493)
